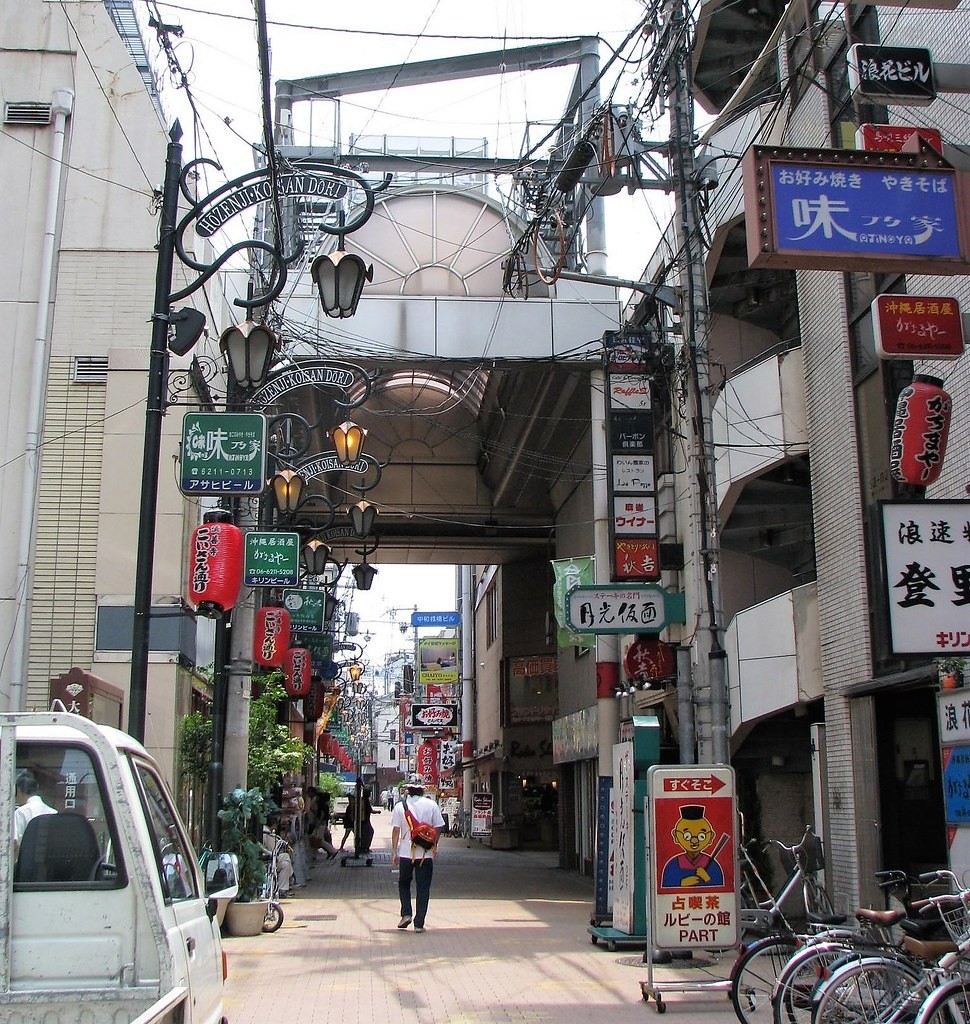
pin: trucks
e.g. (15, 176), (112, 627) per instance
(0, 712), (239, 1024)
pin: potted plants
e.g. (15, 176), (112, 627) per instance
(211, 786), (272, 939)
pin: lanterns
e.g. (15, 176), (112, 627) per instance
(284, 644), (313, 702)
(888, 367), (950, 486)
(416, 737), (439, 788)
(302, 676), (327, 725)
(188, 509), (244, 624)
(251, 599), (293, 666)
(318, 730), (355, 774)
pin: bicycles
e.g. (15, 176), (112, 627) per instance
(451, 806), (480, 839)
(258, 830), (293, 932)
(702, 825), (837, 955)
(729, 868), (970, 1024)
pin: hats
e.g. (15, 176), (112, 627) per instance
(402, 773), (426, 790)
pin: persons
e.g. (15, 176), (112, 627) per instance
(13, 771), (59, 862)
(381, 785), (410, 811)
(262, 813), (295, 900)
(361, 787), (382, 856)
(339, 791), (357, 854)
(391, 774), (446, 933)
(304, 784), (339, 861)
(159, 835), (193, 903)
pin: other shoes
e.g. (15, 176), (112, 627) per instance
(398, 915), (412, 928)
(413, 927), (426, 933)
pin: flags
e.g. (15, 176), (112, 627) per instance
(550, 555), (598, 649)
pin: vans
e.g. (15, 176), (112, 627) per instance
(329, 782), (362, 824)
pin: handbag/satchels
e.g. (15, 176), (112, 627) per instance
(400, 797), (442, 850)
